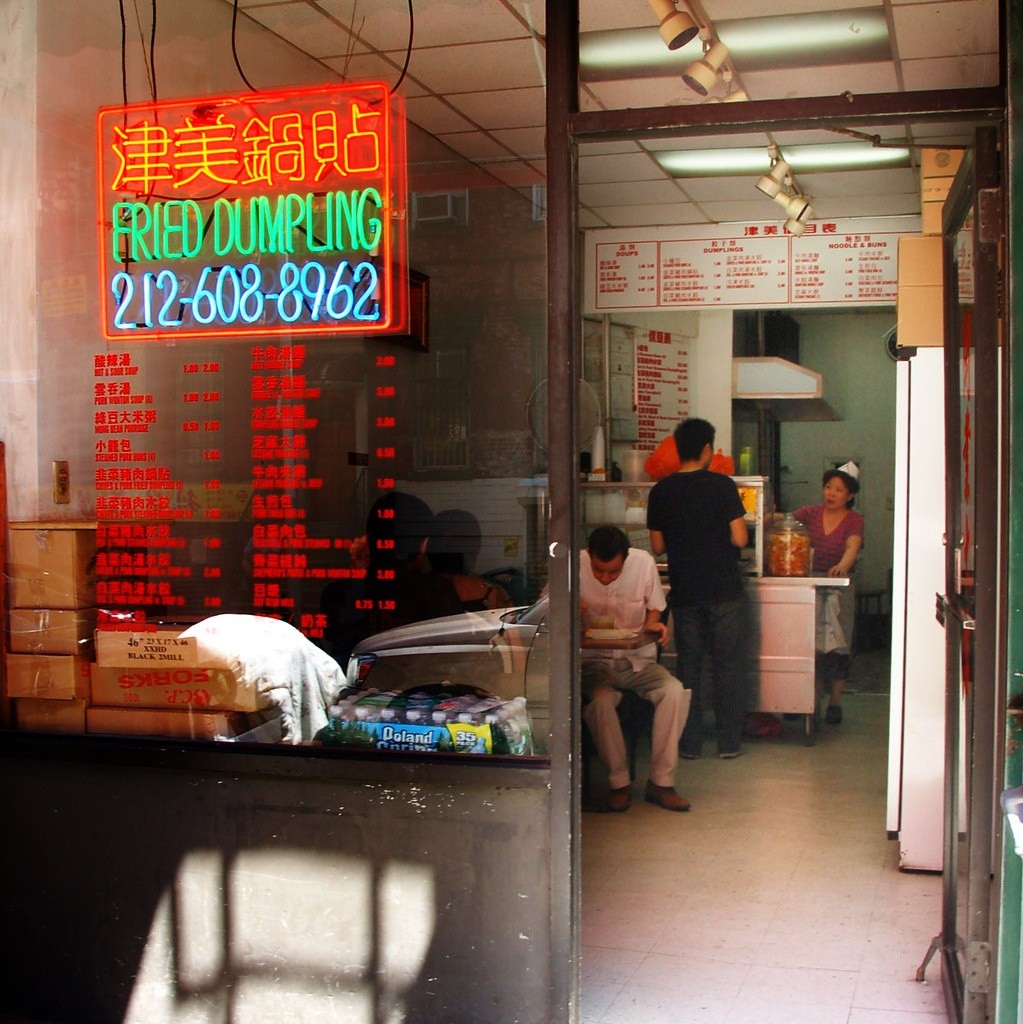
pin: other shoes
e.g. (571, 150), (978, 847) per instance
(719, 747), (747, 758)
(681, 752), (698, 758)
(826, 704), (842, 723)
(783, 712), (800, 720)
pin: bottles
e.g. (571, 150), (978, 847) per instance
(328, 687), (534, 755)
(767, 513), (810, 576)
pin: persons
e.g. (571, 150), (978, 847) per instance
(319, 493), (526, 680)
(764, 460), (865, 726)
(540, 524), (693, 812)
(646, 418), (752, 759)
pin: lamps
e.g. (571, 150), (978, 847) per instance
(701, 71), (748, 103)
(772, 177), (815, 223)
(786, 193), (812, 238)
(679, 28), (730, 96)
(645, 0), (701, 52)
(754, 144), (798, 199)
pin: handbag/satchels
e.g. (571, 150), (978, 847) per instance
(814, 587), (846, 654)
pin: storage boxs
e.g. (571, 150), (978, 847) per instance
(8, 516), (272, 735)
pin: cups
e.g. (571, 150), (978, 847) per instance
(622, 450), (653, 482)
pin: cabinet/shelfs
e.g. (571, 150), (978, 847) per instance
(574, 478), (774, 577)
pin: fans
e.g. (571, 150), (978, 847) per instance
(527, 366), (600, 456)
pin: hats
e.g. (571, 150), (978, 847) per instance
(837, 461), (859, 481)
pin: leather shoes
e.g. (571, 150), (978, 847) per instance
(644, 779), (690, 812)
(608, 784), (633, 811)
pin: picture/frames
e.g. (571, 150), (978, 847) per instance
(369, 255), (429, 355)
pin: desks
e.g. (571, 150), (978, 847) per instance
(580, 628), (664, 651)
(658, 581), (845, 744)
